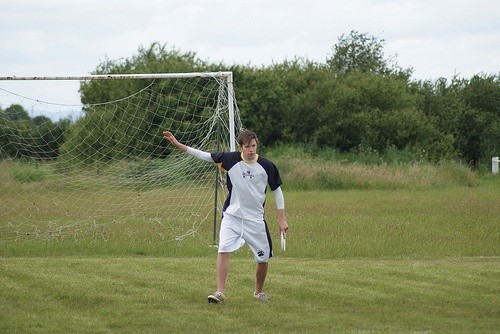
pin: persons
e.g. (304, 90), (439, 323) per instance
(163, 129), (290, 303)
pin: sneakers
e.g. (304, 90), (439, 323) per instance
(208, 291), (225, 304)
(253, 292), (267, 301)
